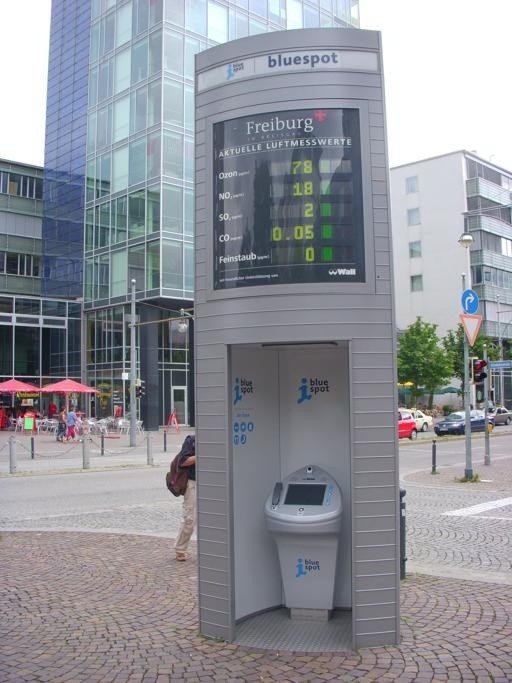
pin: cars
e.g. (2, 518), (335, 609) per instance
(434, 406), (512, 436)
(397, 408), (433, 441)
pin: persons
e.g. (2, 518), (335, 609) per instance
(57, 405), (67, 439)
(7, 413), (14, 429)
(64, 407), (82, 442)
(47, 400), (58, 417)
(115, 404), (123, 418)
(175, 433), (197, 561)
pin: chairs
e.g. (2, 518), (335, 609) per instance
(11, 411), (143, 438)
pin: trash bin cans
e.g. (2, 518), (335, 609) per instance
(400, 488), (408, 580)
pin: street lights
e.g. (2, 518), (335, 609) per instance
(457, 232), (475, 290)
(177, 308), (194, 334)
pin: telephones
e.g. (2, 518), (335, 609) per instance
(272, 482), (282, 505)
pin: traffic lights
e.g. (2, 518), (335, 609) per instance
(473, 360), (487, 403)
(136, 379), (147, 397)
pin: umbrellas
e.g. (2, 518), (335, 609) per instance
(0, 378), (41, 406)
(42, 378), (99, 412)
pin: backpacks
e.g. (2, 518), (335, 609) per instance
(165, 435), (195, 496)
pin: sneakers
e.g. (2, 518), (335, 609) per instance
(175, 552), (187, 561)
